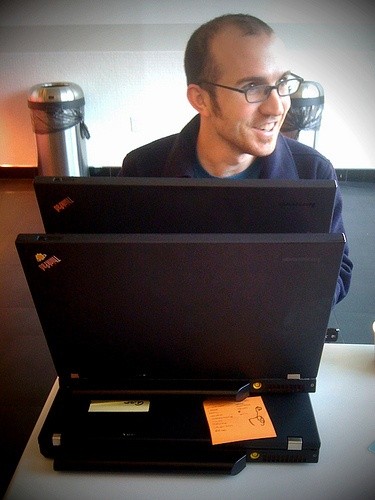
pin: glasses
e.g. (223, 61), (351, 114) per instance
(197, 72), (304, 103)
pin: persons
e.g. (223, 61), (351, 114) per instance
(121, 14), (353, 309)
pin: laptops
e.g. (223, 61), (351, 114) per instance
(36, 391), (322, 476)
(14, 233), (347, 403)
(31, 175), (338, 233)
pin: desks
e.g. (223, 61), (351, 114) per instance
(1, 341), (375, 496)
